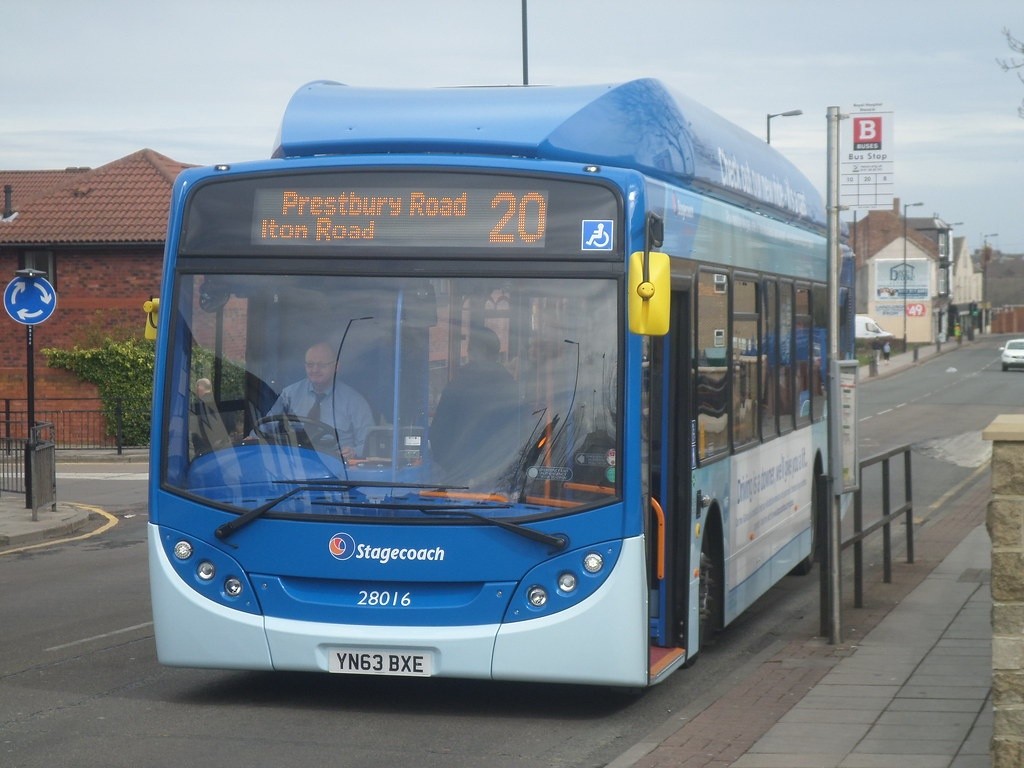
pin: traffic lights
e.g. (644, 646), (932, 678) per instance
(973, 303), (978, 317)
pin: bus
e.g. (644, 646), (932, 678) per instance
(144, 74), (860, 685)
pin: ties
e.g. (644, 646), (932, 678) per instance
(303, 391), (325, 436)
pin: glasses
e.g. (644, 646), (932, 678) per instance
(305, 361), (336, 369)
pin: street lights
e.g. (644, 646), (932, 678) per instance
(766, 109), (803, 143)
(946, 222), (964, 338)
(854, 209), (871, 256)
(903, 201), (923, 352)
(983, 233), (999, 334)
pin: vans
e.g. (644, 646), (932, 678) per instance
(855, 315), (895, 339)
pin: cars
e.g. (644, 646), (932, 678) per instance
(998, 338), (1024, 372)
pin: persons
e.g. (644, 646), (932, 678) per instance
(431, 328), (519, 464)
(954, 323), (961, 341)
(872, 336), (882, 366)
(243, 342), (375, 464)
(882, 341), (891, 363)
